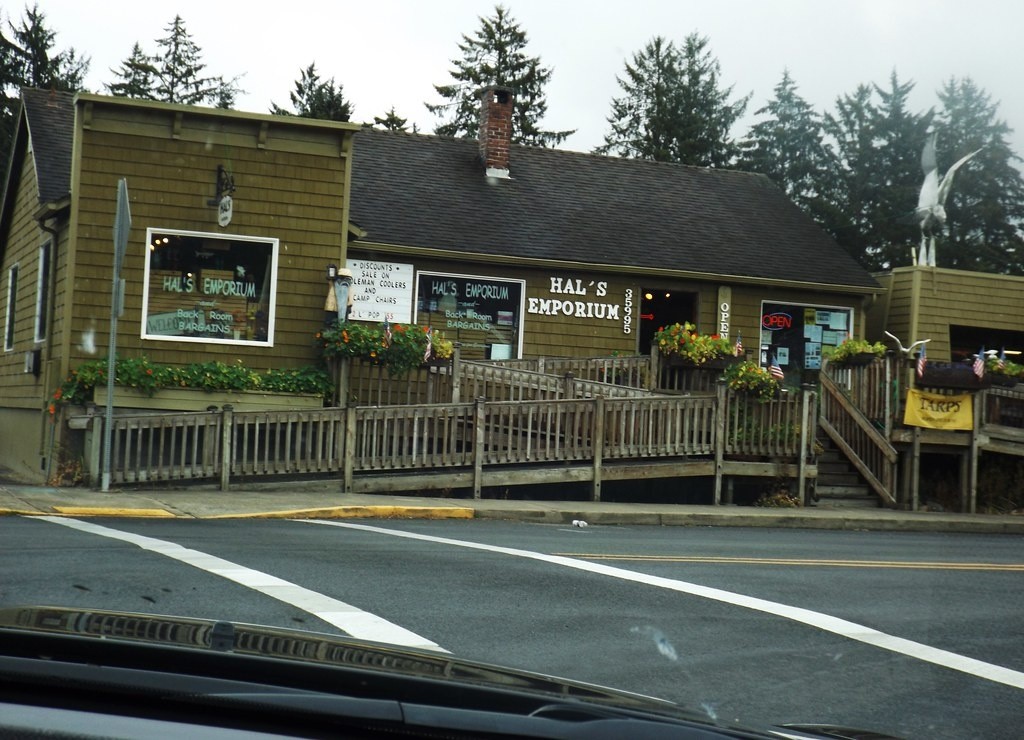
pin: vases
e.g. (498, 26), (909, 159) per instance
(664, 347), (746, 370)
(732, 380), (783, 400)
(92, 385), (324, 413)
(599, 371), (643, 388)
(840, 352), (876, 366)
(988, 371), (1018, 388)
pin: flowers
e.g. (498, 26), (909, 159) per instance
(40, 354), (357, 425)
(649, 321), (736, 367)
(597, 351), (635, 378)
(988, 359), (1024, 375)
(713, 361), (780, 404)
(311, 316), (454, 381)
(729, 402), (809, 445)
(830, 334), (888, 364)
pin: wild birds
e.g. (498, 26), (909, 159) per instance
(983, 350), (998, 355)
(884, 330), (931, 353)
